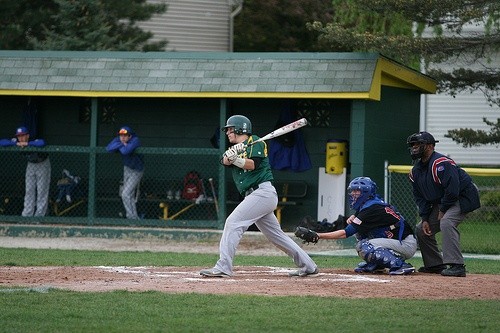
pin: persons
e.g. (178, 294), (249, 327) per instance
(294, 177), (417, 275)
(408, 131), (480, 277)
(105, 126), (144, 219)
(0, 127), (50, 216)
(200, 114), (320, 277)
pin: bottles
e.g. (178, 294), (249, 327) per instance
(175, 191), (181, 200)
(167, 190), (173, 199)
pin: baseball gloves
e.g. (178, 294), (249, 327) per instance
(294, 226), (319, 243)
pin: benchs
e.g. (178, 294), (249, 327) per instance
(55, 196), (296, 225)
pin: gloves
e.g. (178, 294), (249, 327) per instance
(222, 147), (245, 168)
(231, 143), (245, 155)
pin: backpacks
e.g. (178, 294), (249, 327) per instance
(181, 170), (202, 199)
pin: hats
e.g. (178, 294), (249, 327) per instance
(16, 127), (27, 135)
(118, 126), (131, 134)
(407, 132), (439, 146)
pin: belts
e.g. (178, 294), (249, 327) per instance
(245, 185), (259, 195)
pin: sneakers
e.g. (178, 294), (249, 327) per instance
(419, 264), (447, 273)
(289, 267), (320, 276)
(200, 268), (230, 278)
(355, 263), (384, 273)
(441, 265), (466, 276)
(389, 263), (416, 274)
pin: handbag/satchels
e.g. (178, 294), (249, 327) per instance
(302, 215), (348, 234)
(459, 183), (481, 214)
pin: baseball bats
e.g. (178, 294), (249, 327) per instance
(199, 178), (207, 200)
(244, 118), (308, 148)
(209, 178), (218, 212)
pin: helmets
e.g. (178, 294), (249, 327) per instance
(347, 177), (377, 210)
(224, 115), (252, 134)
